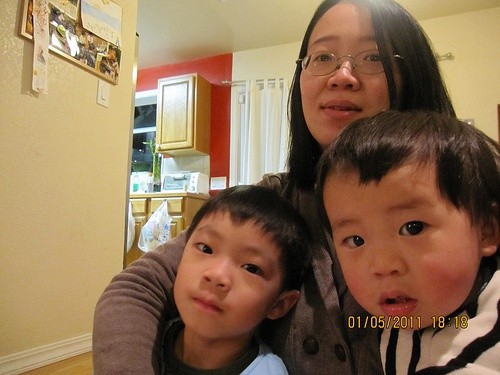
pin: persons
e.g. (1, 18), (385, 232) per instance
(155, 185), (309, 375)
(92, 0), (458, 375)
(314, 110), (500, 375)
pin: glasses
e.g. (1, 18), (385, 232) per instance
(296, 47), (403, 76)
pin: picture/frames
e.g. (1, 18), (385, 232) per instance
(19, 0), (123, 86)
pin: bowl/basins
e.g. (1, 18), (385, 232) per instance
(132, 163), (150, 171)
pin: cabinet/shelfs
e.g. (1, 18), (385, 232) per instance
(154, 72), (212, 156)
(126, 192), (211, 266)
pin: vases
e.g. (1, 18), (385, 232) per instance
(153, 153), (161, 192)
